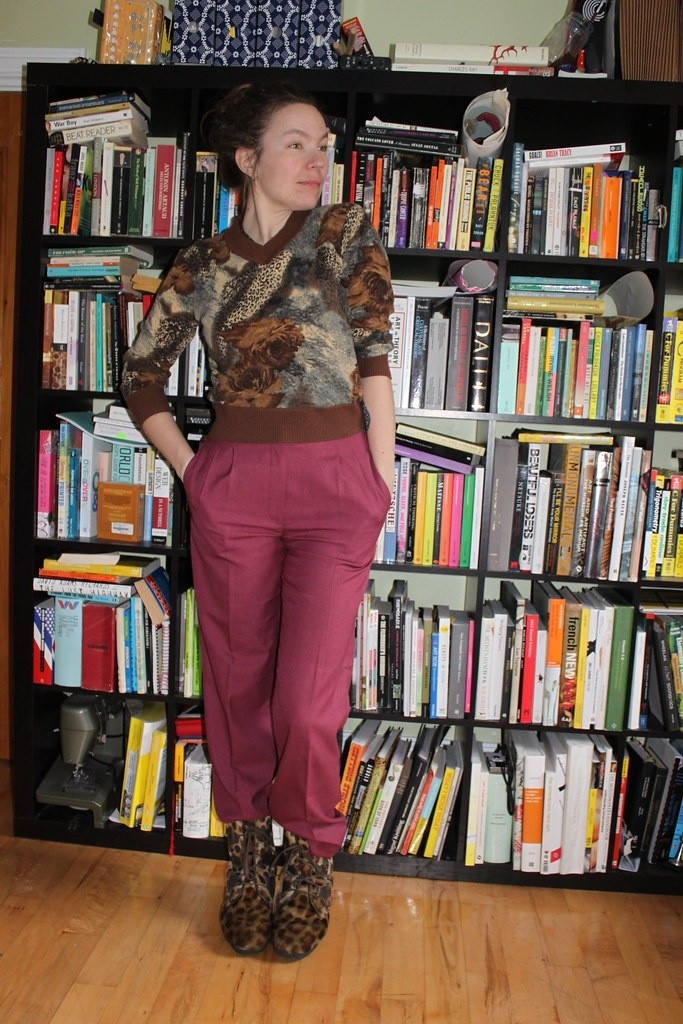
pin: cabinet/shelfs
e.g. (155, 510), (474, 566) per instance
(10, 62), (683, 898)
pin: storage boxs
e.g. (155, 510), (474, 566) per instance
(101, 0), (343, 67)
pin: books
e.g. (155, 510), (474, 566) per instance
(42, 89), (346, 240)
(354, 115), (504, 253)
(32, 551), (203, 700)
(387, 279), (495, 412)
(374, 422), (486, 570)
(349, 578), (475, 720)
(473, 579), (635, 732)
(496, 275), (683, 425)
(627, 586), (683, 732)
(36, 398), (177, 550)
(486, 427), (683, 583)
(507, 130), (683, 263)
(42, 243), (210, 398)
(119, 81), (396, 960)
(117, 701), (464, 862)
(464, 726), (683, 876)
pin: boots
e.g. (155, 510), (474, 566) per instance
(219, 815), (278, 954)
(272, 829), (334, 959)
